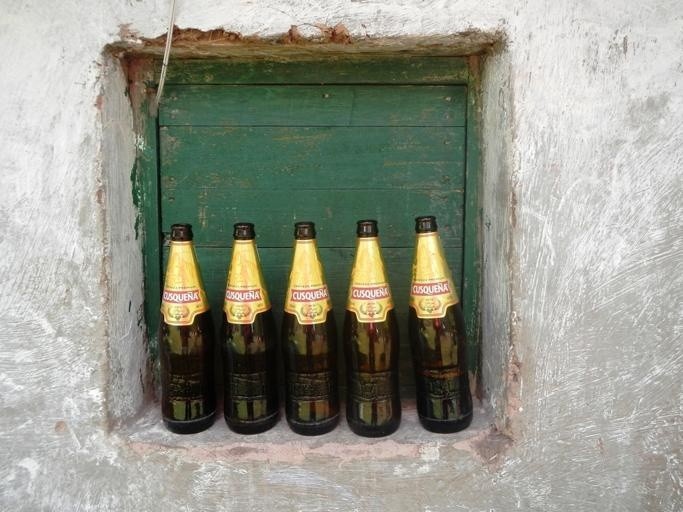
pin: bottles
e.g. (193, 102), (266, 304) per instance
(158, 215), (224, 435)
(223, 215), (282, 435)
(407, 213), (476, 433)
(342, 217), (402, 438)
(282, 217), (342, 436)
